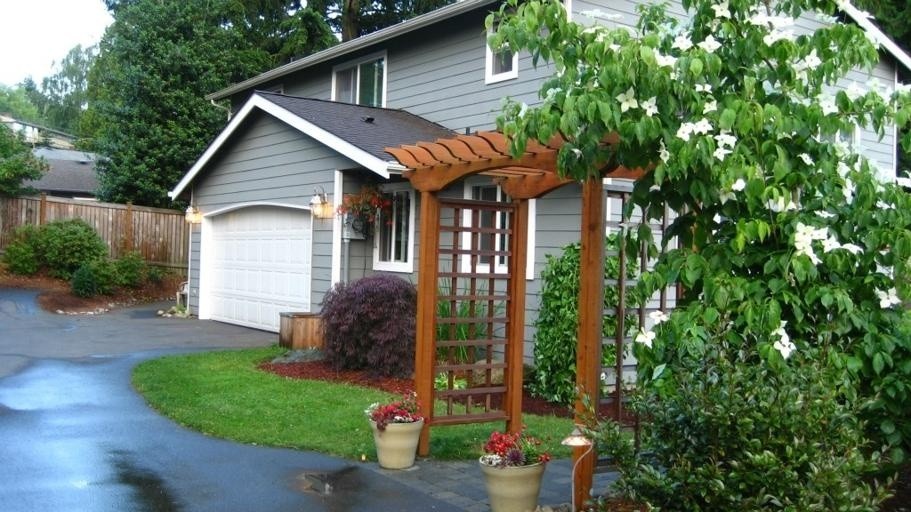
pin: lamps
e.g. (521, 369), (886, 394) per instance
(560, 427), (595, 512)
(309, 185), (328, 219)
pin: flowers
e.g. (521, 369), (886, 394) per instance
(364, 386), (424, 431)
(334, 188), (402, 233)
(478, 430), (552, 472)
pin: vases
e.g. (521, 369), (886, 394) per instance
(478, 455), (544, 512)
(367, 417), (423, 471)
